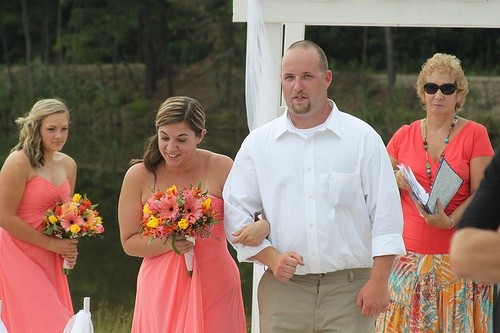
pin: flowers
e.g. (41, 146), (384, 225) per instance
(138, 180), (221, 277)
(41, 192), (105, 277)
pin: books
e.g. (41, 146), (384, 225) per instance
(396, 157), (464, 215)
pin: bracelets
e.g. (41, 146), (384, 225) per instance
(448, 218), (454, 229)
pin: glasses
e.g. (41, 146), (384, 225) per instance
(424, 82), (457, 95)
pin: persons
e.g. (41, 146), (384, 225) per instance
(387, 54), (495, 333)
(220, 40), (408, 332)
(0, 97), (79, 333)
(118, 95), (270, 333)
(450, 151), (500, 333)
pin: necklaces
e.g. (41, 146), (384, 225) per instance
(422, 114), (457, 192)
